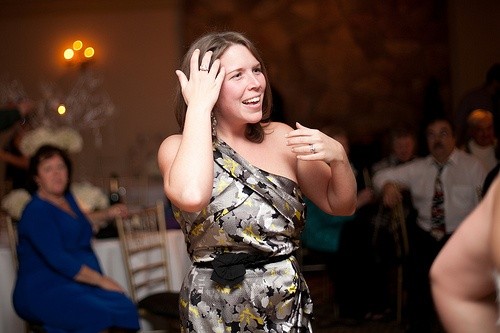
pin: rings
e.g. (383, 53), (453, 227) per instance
(200, 65), (209, 71)
(310, 145), (315, 153)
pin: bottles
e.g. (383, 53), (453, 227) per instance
(109, 173), (120, 206)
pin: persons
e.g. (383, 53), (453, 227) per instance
(428, 172), (500, 333)
(159, 32), (357, 333)
(13, 144), (141, 333)
(297, 107), (500, 333)
(0, 97), (36, 193)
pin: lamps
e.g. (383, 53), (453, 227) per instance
(63, 39), (98, 78)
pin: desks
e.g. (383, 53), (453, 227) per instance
(93, 229), (193, 301)
(0, 247), (27, 333)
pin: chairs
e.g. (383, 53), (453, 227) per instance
(5, 213), (20, 273)
(388, 203), (409, 323)
(115, 198), (183, 333)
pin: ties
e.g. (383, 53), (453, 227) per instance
(431, 161), (446, 242)
